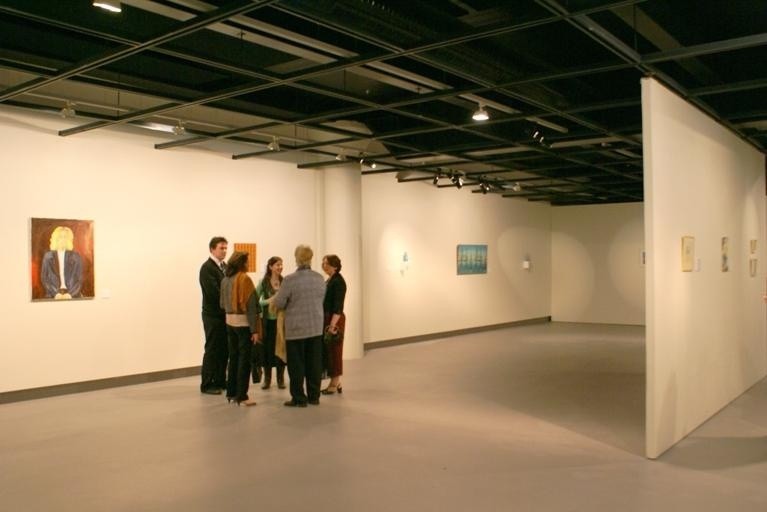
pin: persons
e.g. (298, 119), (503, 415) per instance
(256, 257), (286, 390)
(320, 255), (346, 394)
(220, 251), (262, 406)
(199, 237), (228, 394)
(274, 246), (326, 407)
(41, 226), (83, 299)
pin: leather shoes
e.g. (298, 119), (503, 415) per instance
(201, 369), (342, 407)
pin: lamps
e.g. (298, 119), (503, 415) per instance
(472, 104), (489, 120)
(92, 0), (122, 13)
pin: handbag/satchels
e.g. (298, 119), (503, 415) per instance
(324, 331), (340, 345)
